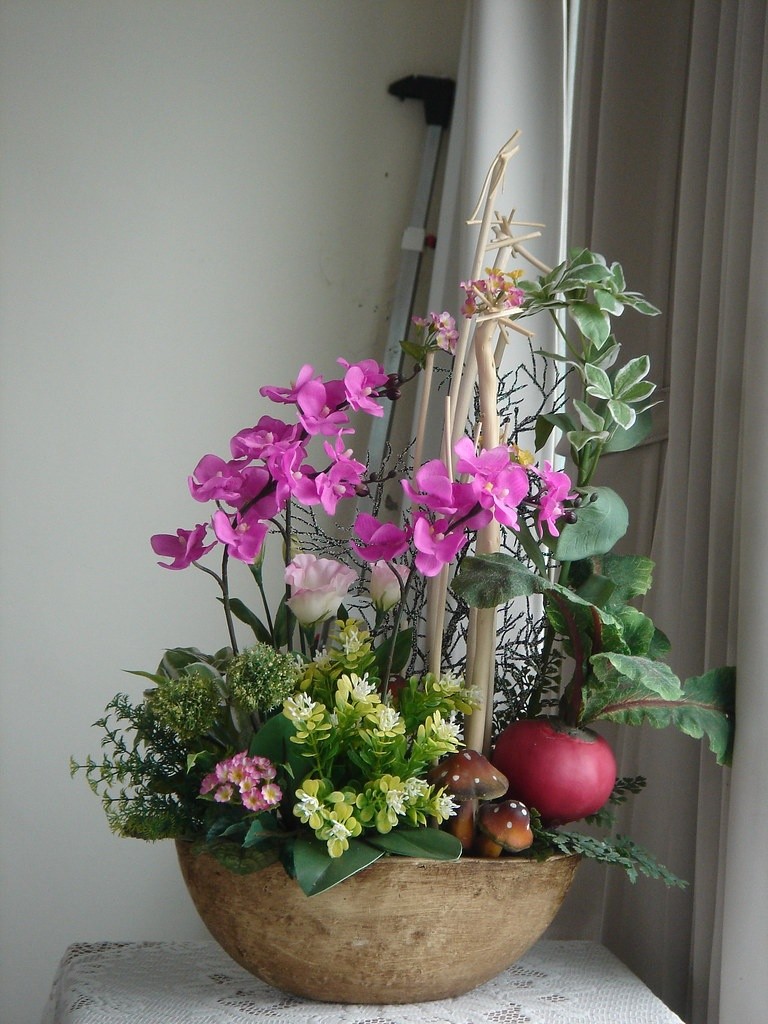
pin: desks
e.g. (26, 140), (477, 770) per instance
(27, 938), (685, 1024)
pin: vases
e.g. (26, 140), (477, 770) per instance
(171, 836), (587, 1007)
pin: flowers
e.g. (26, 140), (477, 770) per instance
(72, 243), (738, 895)
